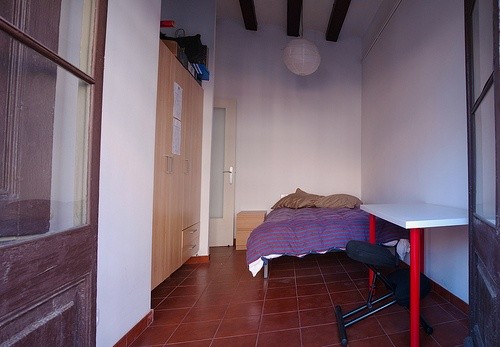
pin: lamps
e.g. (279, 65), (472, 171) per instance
(283, 0), (320, 76)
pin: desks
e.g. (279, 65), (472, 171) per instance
(360, 202), (468, 347)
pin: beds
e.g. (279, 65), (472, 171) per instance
(246, 189), (410, 278)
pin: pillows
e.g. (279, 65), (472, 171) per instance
(271, 188), (362, 208)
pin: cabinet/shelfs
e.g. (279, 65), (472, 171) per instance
(151, 37), (204, 291)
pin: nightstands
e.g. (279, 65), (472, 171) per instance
(236, 211), (267, 250)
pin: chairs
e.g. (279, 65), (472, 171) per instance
(335, 240), (433, 347)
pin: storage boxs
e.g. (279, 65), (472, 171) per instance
(163, 40), (188, 69)
